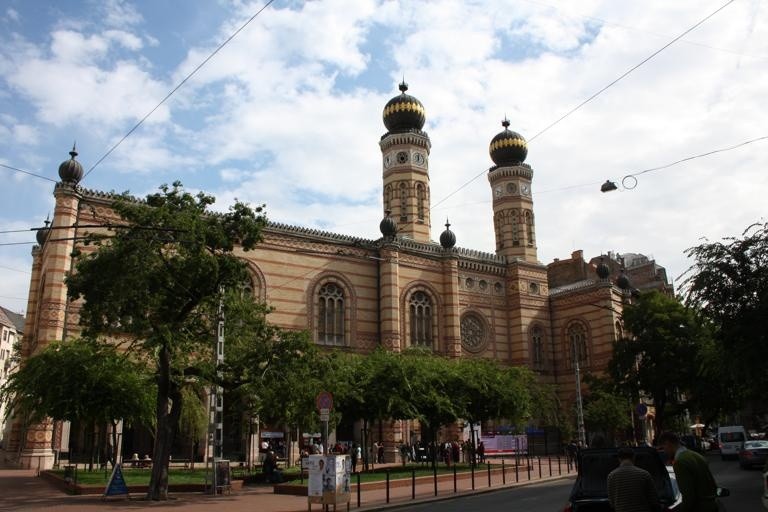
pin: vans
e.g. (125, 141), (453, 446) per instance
(718, 426), (748, 461)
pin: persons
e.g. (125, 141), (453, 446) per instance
(660, 431), (718, 511)
(261, 449), (282, 483)
(566, 428), (718, 471)
(144, 454), (152, 467)
(341, 456), (351, 492)
(131, 452), (139, 466)
(607, 446), (665, 511)
(262, 437), (486, 466)
(317, 456), (336, 491)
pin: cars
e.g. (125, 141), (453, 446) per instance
(562, 439), (768, 512)
